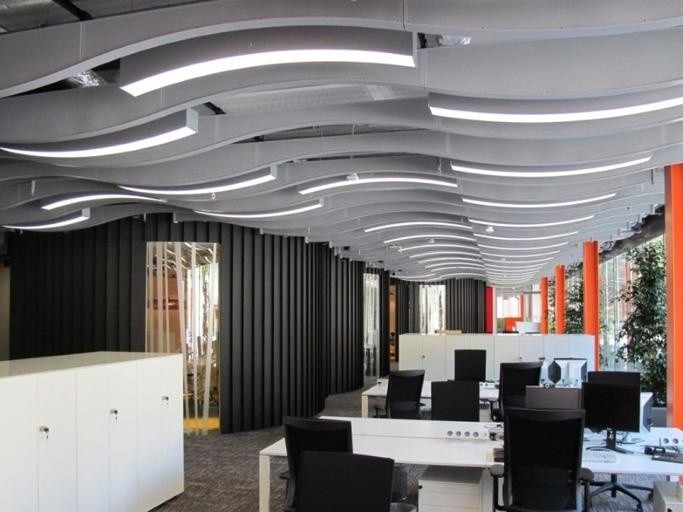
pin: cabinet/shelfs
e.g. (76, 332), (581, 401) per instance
(78, 360), (139, 512)
(543, 332), (595, 387)
(447, 333), (495, 383)
(139, 354), (185, 512)
(495, 333), (543, 381)
(397, 332), (447, 382)
(0, 363), (78, 512)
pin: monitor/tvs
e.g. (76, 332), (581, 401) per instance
(526, 386), (581, 410)
(581, 382), (640, 455)
